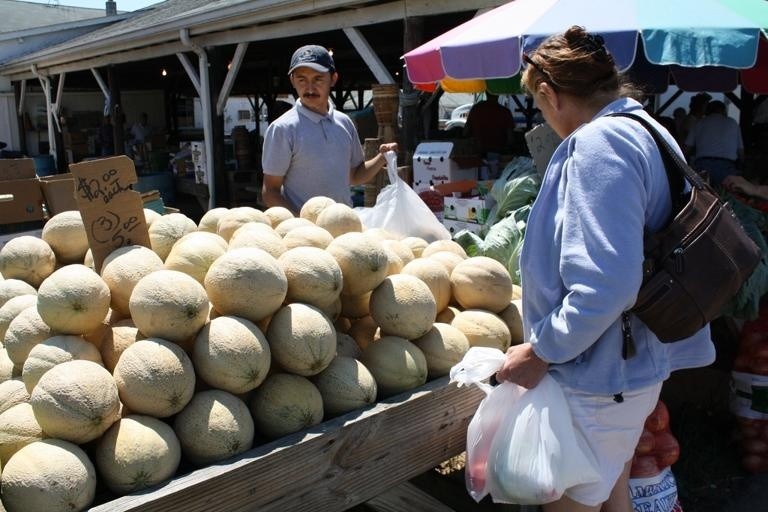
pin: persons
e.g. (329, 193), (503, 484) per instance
(98, 115), (115, 157)
(462, 90), (516, 160)
(260, 45), (398, 218)
(496, 25), (716, 512)
(129, 112), (154, 141)
(643, 91), (768, 189)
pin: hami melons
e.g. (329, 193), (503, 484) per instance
(0, 196), (523, 512)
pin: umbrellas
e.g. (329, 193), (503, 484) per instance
(400, 0), (768, 96)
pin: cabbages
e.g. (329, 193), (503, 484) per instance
(454, 171), (541, 286)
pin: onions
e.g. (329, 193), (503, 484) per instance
(629, 400), (680, 479)
(733, 320), (768, 473)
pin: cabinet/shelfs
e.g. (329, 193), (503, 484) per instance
(87, 374), (487, 512)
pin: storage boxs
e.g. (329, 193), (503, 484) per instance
(191, 136), (227, 163)
(0, 158), (79, 224)
(64, 131), (88, 163)
(194, 163), (224, 184)
(412, 142), (496, 237)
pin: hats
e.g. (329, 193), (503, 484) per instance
(288, 44), (336, 73)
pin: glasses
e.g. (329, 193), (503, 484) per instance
(522, 49), (560, 92)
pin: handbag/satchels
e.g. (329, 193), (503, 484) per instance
(631, 183), (762, 343)
